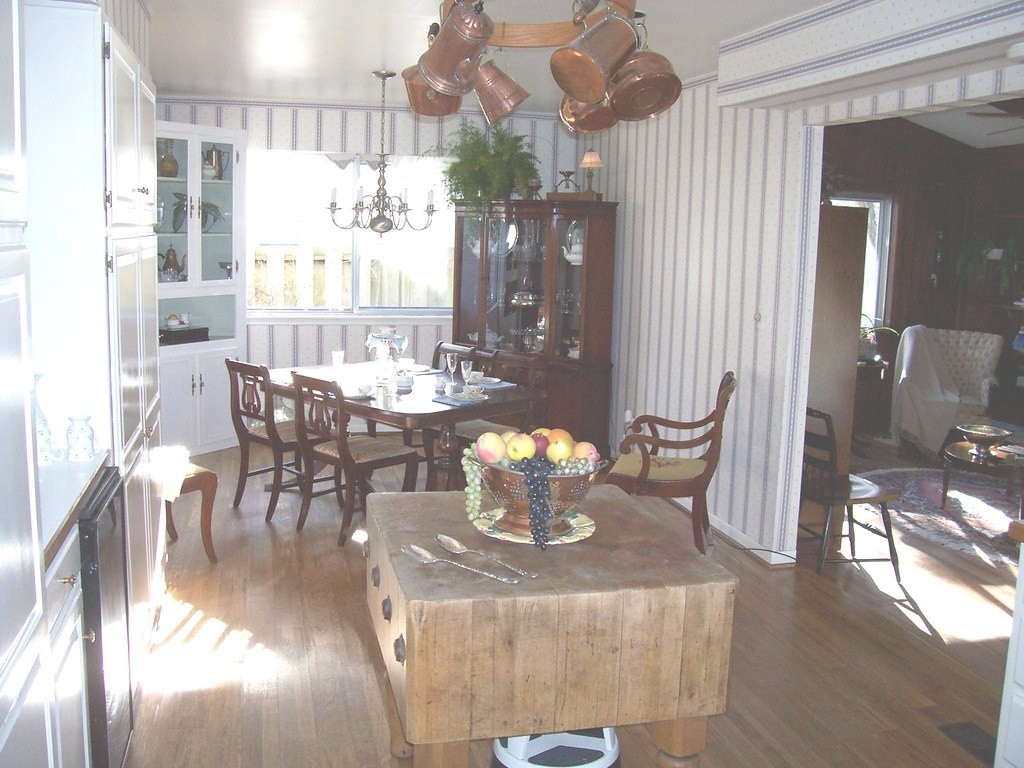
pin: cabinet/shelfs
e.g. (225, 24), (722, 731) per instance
(451, 199), (620, 469)
(955, 194), (1024, 427)
(28, 229), (161, 476)
(122, 421), (165, 729)
(158, 346), (247, 457)
(22, 0), (158, 228)
(0, 520), (93, 768)
(156, 120), (247, 346)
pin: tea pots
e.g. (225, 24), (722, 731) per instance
(467, 324), (505, 348)
(202, 142), (230, 180)
(157, 244), (186, 274)
(560, 244), (582, 264)
(468, 235), (500, 257)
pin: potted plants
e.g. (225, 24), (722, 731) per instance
(417, 116), (542, 244)
(954, 222), (1022, 296)
(857, 313), (900, 361)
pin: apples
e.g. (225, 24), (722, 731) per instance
(477, 427), (599, 464)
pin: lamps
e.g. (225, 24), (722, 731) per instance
(579, 147), (605, 193)
(326, 70), (438, 239)
(401, 0), (682, 136)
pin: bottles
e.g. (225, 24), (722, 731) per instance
(158, 139), (179, 176)
(436, 377), (446, 390)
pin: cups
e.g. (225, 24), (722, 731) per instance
(470, 371), (484, 382)
(168, 268), (178, 282)
(202, 160), (218, 179)
(332, 351), (344, 370)
(397, 358), (415, 369)
(158, 268), (169, 283)
(463, 384), (483, 398)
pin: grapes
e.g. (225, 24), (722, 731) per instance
(551, 456), (599, 476)
(509, 457), (556, 551)
(461, 443), (483, 522)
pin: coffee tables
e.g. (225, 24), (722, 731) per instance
(939, 441), (1024, 521)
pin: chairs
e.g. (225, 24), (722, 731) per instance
(422, 350), (542, 492)
(797, 407), (902, 583)
(165, 461), (217, 564)
(605, 370), (737, 555)
(224, 357), (349, 523)
(366, 340), (475, 479)
(290, 369), (418, 547)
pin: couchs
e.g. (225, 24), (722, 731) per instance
(891, 324), (1005, 464)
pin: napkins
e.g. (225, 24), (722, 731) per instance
(411, 368), (443, 375)
(478, 381), (517, 391)
(431, 396), (474, 407)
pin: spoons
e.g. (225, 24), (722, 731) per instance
(400, 544), (521, 585)
(435, 534), (537, 578)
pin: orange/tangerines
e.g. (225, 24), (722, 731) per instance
(547, 429), (572, 442)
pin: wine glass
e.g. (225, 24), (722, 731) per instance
(447, 353), (458, 386)
(461, 361), (472, 394)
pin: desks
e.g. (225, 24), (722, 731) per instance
(362, 483), (739, 768)
(852, 359), (889, 422)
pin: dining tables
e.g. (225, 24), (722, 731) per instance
(241, 359), (548, 492)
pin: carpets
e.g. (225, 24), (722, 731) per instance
(843, 468), (1024, 567)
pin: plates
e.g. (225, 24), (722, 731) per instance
(473, 508), (596, 543)
(449, 393), (489, 401)
(490, 218), (519, 256)
(342, 392), (375, 398)
(478, 377), (501, 383)
(409, 364), (430, 372)
(565, 219), (586, 250)
